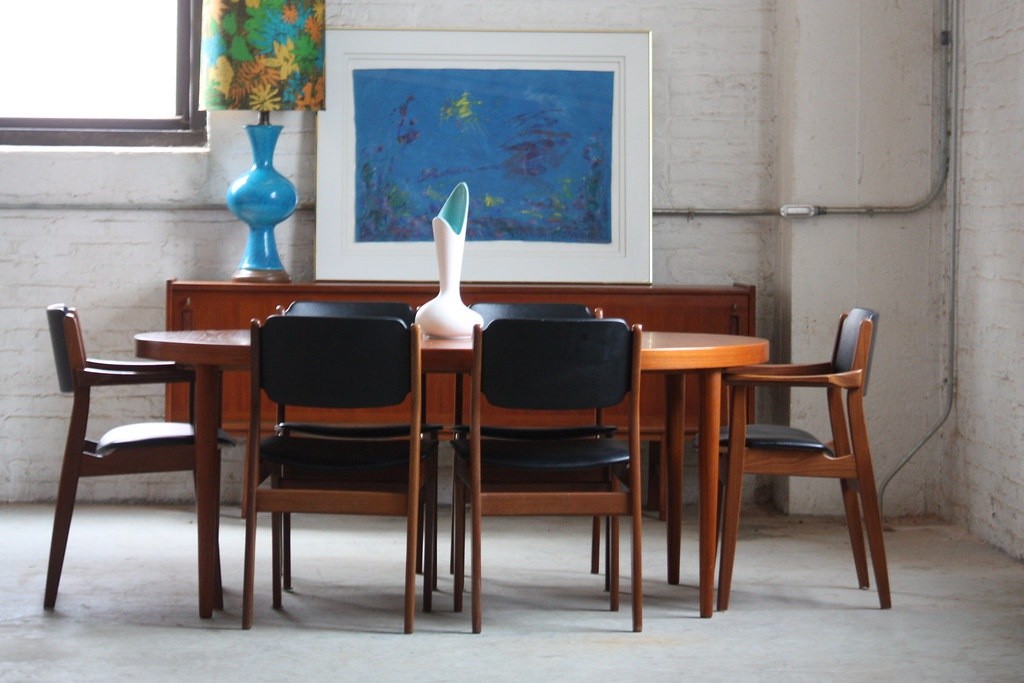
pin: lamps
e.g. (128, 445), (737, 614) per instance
(196, 0), (332, 282)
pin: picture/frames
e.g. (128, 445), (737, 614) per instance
(312, 22), (659, 287)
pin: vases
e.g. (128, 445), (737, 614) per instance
(417, 175), (486, 340)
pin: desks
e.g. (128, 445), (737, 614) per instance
(128, 324), (771, 618)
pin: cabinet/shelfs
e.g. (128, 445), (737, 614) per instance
(153, 273), (768, 525)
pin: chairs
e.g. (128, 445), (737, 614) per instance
(712, 307), (897, 616)
(451, 314), (654, 637)
(450, 296), (633, 593)
(233, 313), (445, 636)
(274, 294), (437, 596)
(40, 305), (220, 620)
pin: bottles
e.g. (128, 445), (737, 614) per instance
(414, 182), (484, 340)
